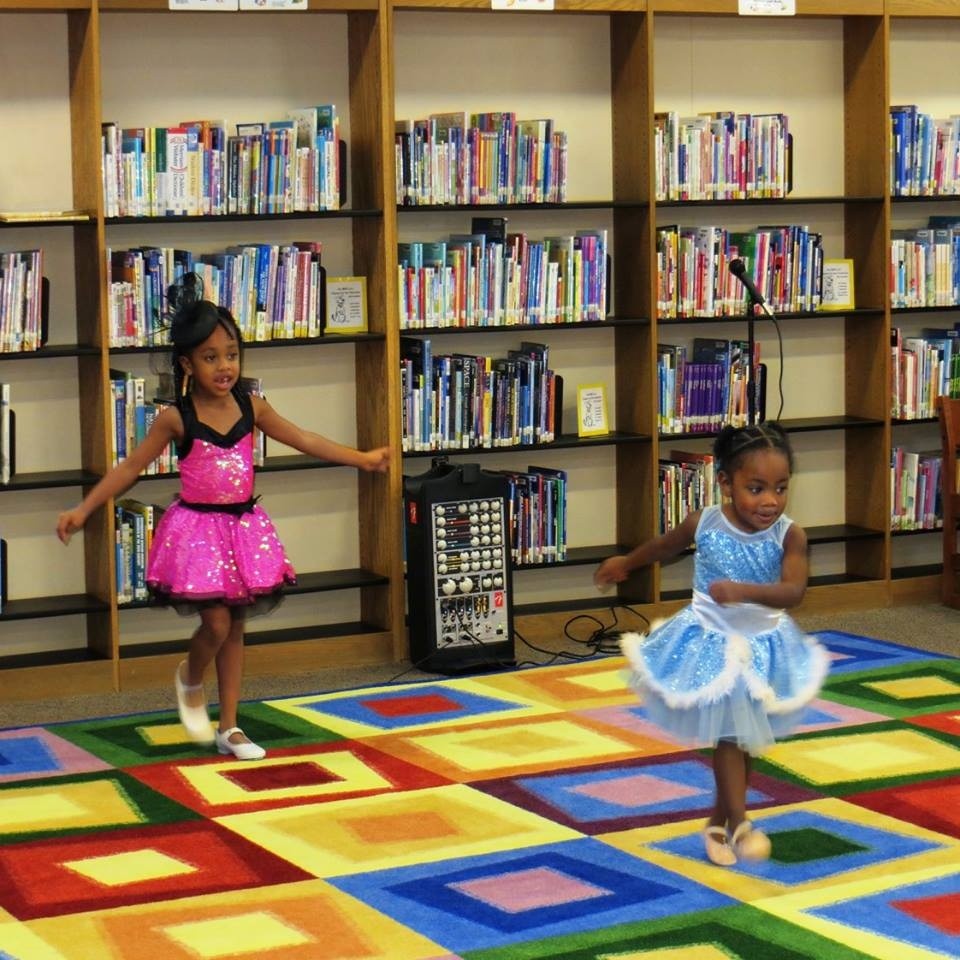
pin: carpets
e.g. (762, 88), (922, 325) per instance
(0, 630), (959, 960)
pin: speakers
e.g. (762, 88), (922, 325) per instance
(404, 463), (517, 675)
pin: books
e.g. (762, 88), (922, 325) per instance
(109, 366), (269, 478)
(106, 233), (370, 348)
(655, 107), (857, 551)
(1, 247), (49, 353)
(100, 103), (348, 217)
(2, 206), (93, 223)
(1, 384), (13, 486)
(397, 112), (612, 565)
(889, 103), (960, 531)
(114, 497), (188, 609)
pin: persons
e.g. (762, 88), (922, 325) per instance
(56, 299), (393, 758)
(591, 421), (809, 867)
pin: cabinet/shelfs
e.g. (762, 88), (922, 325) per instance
(0, 1), (959, 705)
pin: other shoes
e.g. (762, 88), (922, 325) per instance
(704, 820), (737, 866)
(215, 727), (266, 759)
(725, 820), (770, 858)
(177, 660), (209, 731)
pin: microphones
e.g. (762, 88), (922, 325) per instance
(730, 259), (774, 316)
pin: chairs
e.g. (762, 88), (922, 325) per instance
(934, 396), (960, 610)
